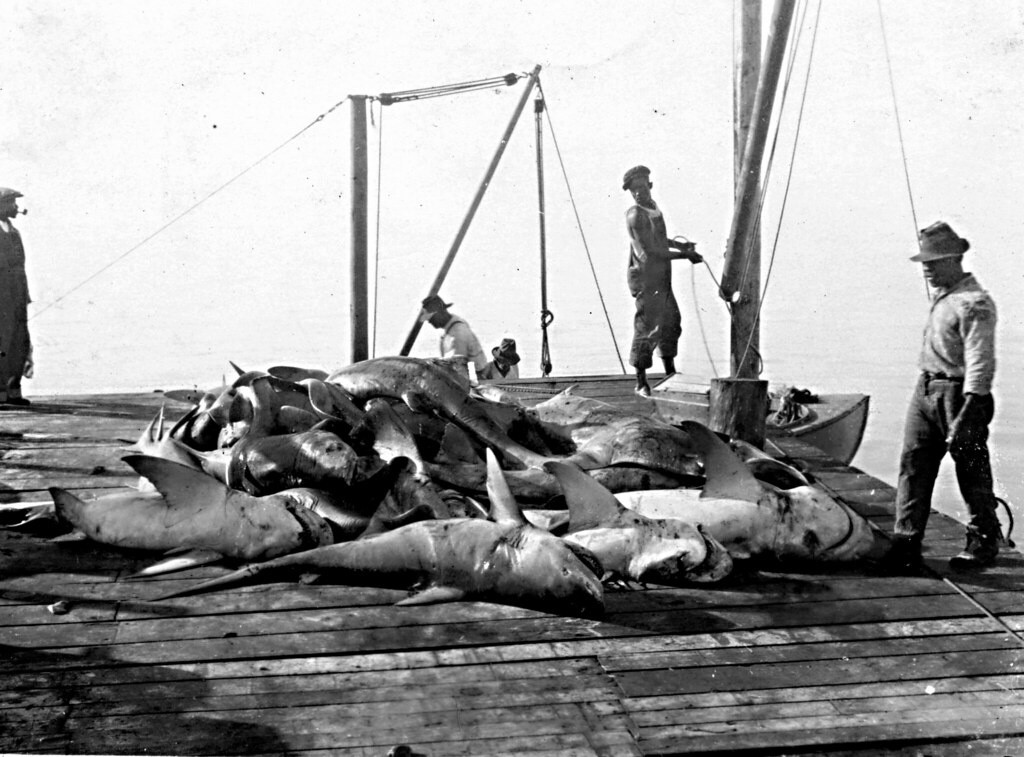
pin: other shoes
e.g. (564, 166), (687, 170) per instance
(862, 540), (924, 573)
(949, 531), (999, 570)
(634, 382), (650, 401)
(0, 397), (30, 407)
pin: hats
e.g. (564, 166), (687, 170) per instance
(419, 294), (452, 321)
(492, 339), (520, 365)
(910, 222), (970, 261)
(0, 190), (24, 201)
(623, 166), (650, 190)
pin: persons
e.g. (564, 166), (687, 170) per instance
(418, 294), (488, 380)
(888, 220), (1001, 575)
(478, 338), (522, 380)
(622, 166), (703, 397)
(0, 186), (34, 409)
